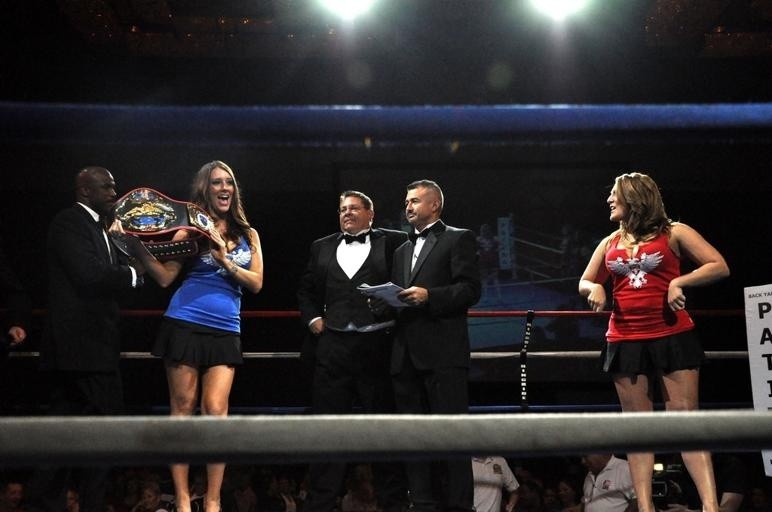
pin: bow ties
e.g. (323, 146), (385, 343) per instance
(408, 229), (428, 243)
(343, 234), (366, 243)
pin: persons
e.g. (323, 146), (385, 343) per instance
(0, 319), (27, 346)
(0, 453), (772, 512)
(365, 179), (481, 512)
(299, 189), (409, 511)
(107, 159), (265, 512)
(578, 172), (731, 512)
(39, 165), (147, 512)
